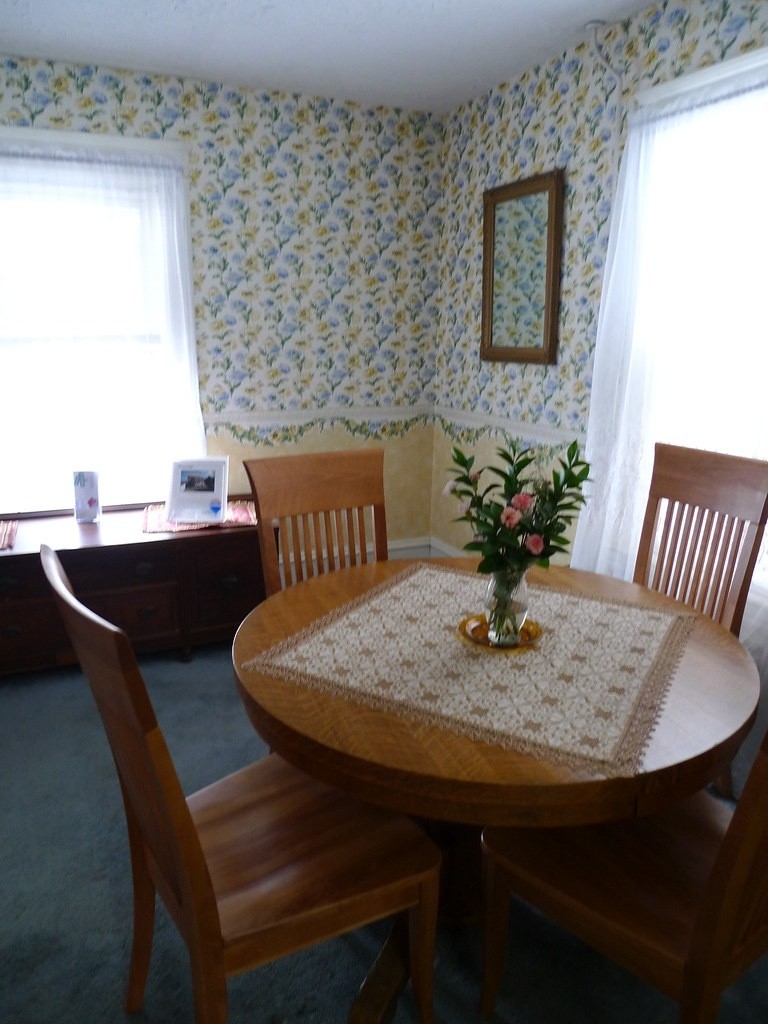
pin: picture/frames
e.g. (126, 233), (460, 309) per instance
(168, 459), (228, 524)
(481, 169), (566, 367)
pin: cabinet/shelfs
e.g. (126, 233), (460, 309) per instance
(0, 501), (280, 657)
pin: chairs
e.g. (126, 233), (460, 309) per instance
(631, 441), (767, 798)
(38, 541), (445, 1024)
(243, 445), (390, 601)
(478, 731), (768, 1024)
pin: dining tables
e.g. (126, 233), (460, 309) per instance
(230, 559), (761, 971)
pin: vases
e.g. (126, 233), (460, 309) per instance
(482, 568), (531, 650)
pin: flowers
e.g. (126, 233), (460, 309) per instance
(442, 430), (594, 577)
